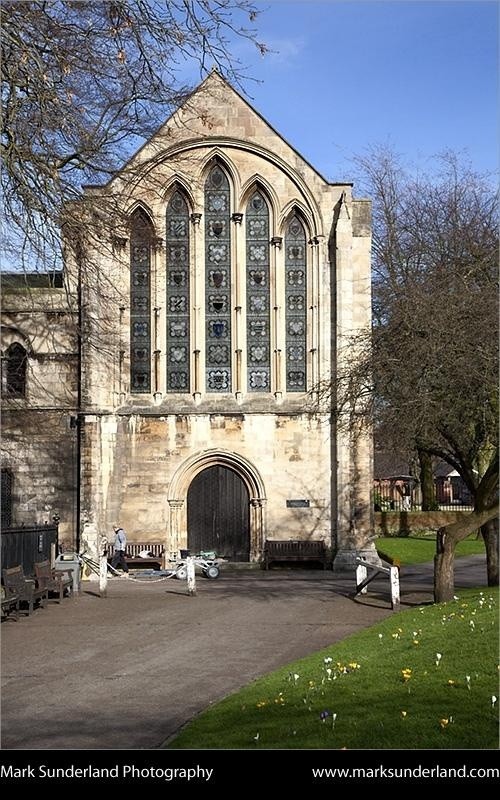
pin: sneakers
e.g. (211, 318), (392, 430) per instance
(107, 573), (113, 578)
(120, 572), (129, 578)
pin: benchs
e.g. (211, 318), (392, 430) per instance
(34, 561), (75, 604)
(2, 564), (48, 616)
(263, 539), (328, 571)
(105, 541), (166, 572)
(1, 585), (19, 621)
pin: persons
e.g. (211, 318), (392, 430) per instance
(106, 524), (129, 579)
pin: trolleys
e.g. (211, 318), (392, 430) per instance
(169, 556), (228, 581)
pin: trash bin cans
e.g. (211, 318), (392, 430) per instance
(55, 553), (82, 592)
